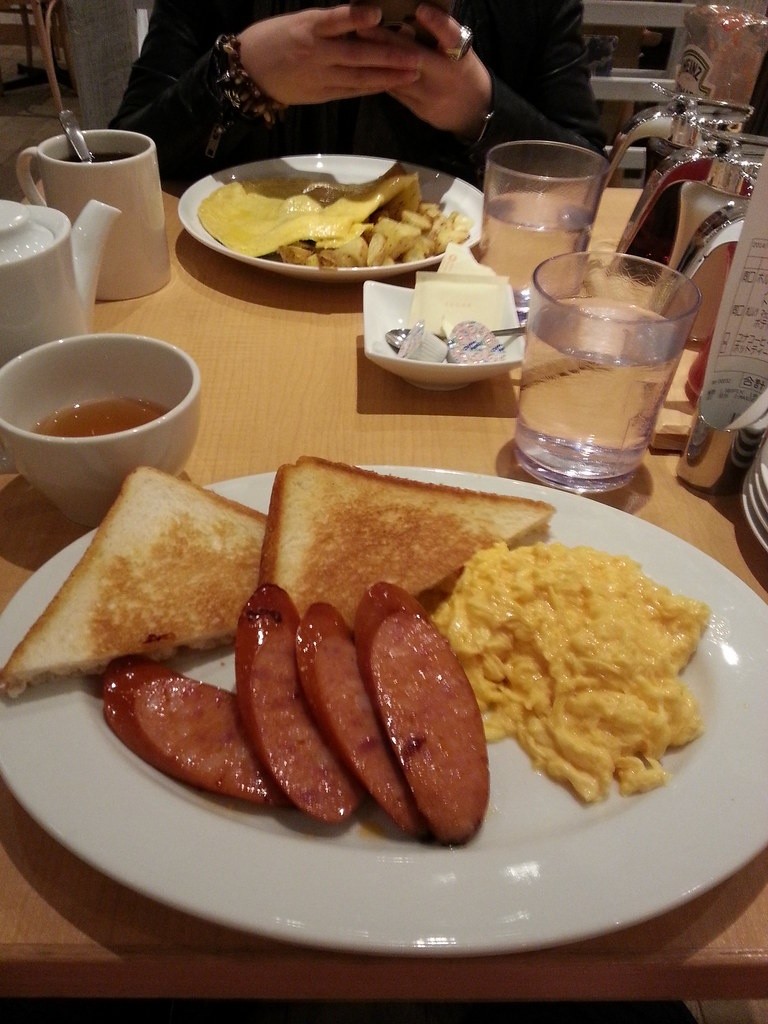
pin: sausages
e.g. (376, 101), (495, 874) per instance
(102, 579), (491, 845)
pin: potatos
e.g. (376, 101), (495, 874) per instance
(277, 203), (471, 270)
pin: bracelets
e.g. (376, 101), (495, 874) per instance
(213, 35), (284, 120)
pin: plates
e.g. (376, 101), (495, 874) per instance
(177, 153), (484, 285)
(0, 464), (768, 958)
(741, 438), (768, 552)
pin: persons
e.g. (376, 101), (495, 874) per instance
(107, 0), (610, 190)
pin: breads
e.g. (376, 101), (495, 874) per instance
(257, 455), (555, 630)
(0, 464), (269, 700)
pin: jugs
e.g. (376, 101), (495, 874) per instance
(603, 81), (768, 353)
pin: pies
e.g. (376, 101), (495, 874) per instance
(198, 162), (418, 258)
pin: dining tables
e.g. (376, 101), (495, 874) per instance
(0, 175), (767, 1000)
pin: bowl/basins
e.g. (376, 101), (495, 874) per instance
(362, 279), (527, 392)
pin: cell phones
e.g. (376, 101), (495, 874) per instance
(346, 0), (455, 50)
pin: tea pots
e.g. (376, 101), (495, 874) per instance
(0, 197), (122, 367)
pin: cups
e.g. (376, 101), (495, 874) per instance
(15, 127), (171, 302)
(512, 250), (702, 495)
(0, 331), (203, 526)
(478, 139), (612, 327)
(676, 392), (768, 496)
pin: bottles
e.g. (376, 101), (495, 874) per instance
(674, 0), (768, 106)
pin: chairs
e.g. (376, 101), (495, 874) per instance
(25, 0), (155, 128)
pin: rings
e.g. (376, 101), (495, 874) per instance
(446, 26), (474, 59)
